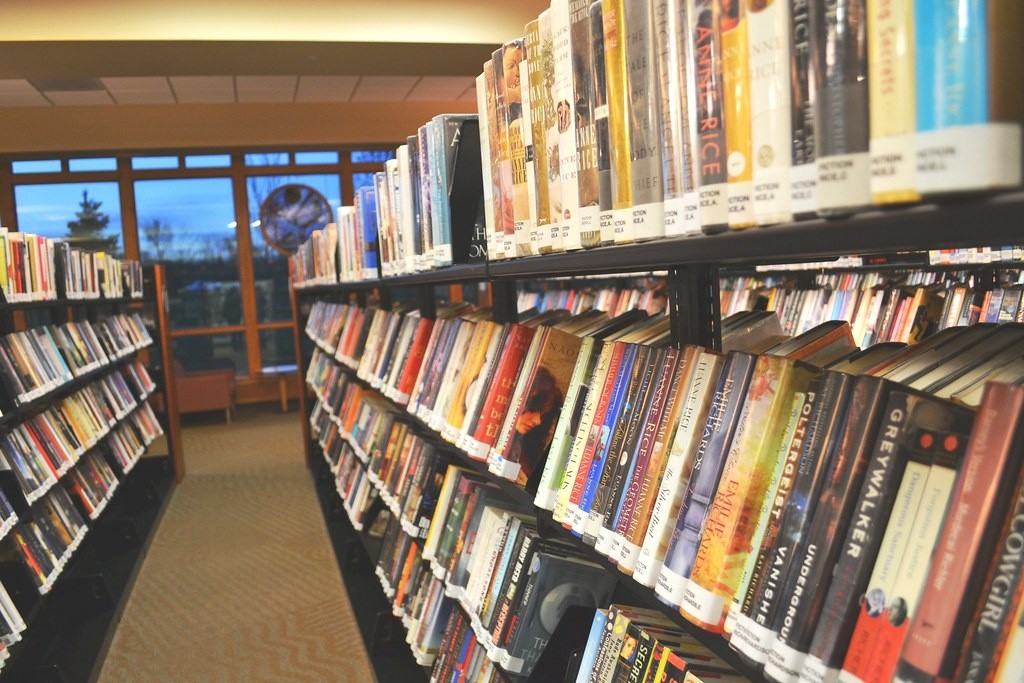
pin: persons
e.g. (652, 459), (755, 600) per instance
(866, 588), (884, 617)
(887, 597), (907, 627)
(507, 365), (562, 466)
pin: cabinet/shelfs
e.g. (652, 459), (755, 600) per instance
(0, 242), (173, 683)
(279, 117), (1024, 683)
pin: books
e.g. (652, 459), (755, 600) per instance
(288, 0), (1024, 683)
(0, 226), (165, 678)
